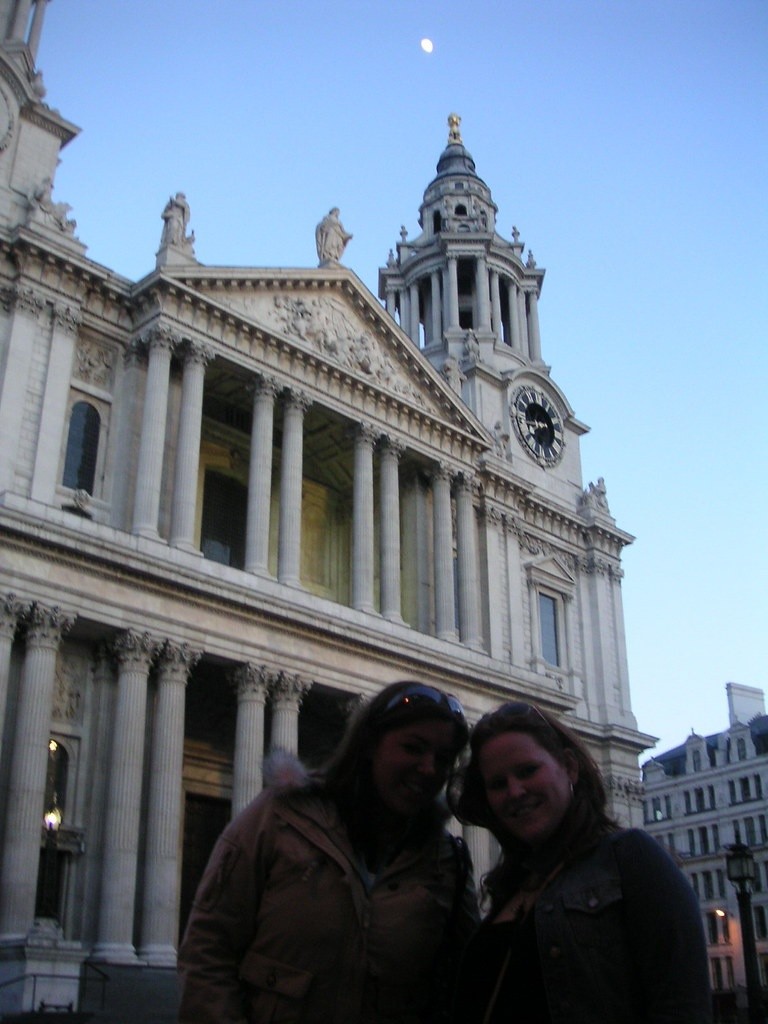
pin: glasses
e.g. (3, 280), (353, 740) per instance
(381, 685), (461, 717)
(472, 701), (560, 740)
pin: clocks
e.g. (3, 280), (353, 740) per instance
(513, 388), (566, 467)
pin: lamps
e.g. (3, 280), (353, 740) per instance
(44, 800), (62, 854)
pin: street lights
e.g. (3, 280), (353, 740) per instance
(726, 831), (768, 1024)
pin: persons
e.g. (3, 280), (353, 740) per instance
(317, 205), (352, 262)
(445, 698), (711, 1024)
(177, 682), (483, 1023)
(586, 476), (610, 514)
(160, 191), (191, 248)
(288, 297), (397, 384)
(489, 419), (509, 460)
(443, 359), (467, 397)
